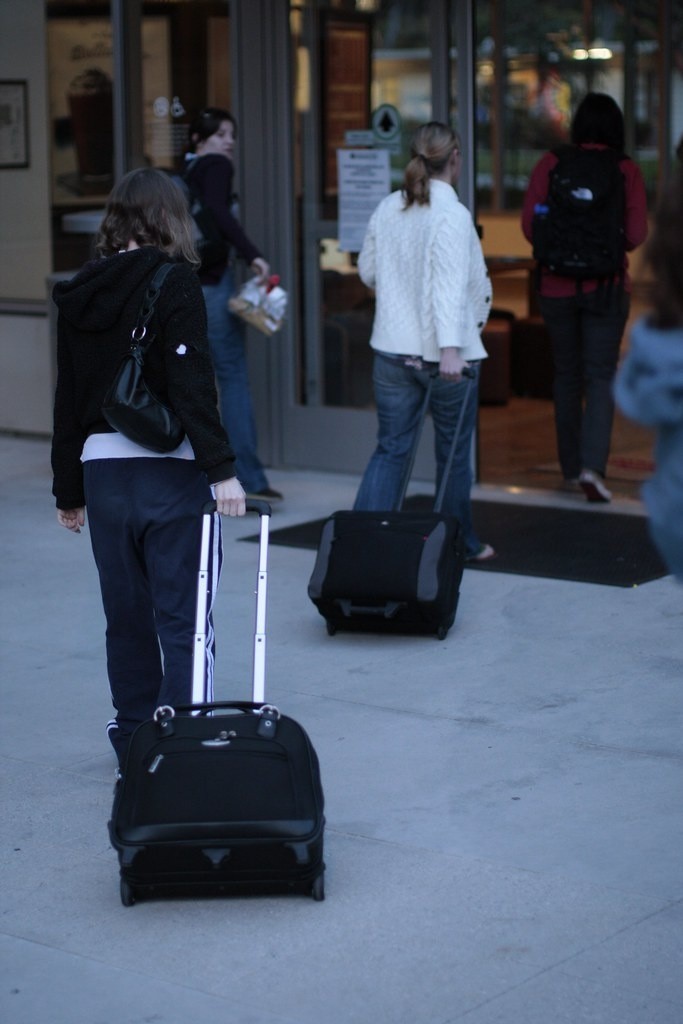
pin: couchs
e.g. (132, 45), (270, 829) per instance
(323, 270), (374, 407)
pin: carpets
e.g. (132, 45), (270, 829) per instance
(236, 494), (675, 589)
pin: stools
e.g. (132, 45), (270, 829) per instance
(479, 308), (555, 404)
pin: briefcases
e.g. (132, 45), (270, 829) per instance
(308, 367), (476, 640)
(107, 497), (326, 906)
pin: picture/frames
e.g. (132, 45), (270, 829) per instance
(0, 78), (31, 170)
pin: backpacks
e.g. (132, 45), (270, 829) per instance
(531, 144), (624, 281)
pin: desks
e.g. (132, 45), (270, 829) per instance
(62, 208), (109, 273)
(483, 255), (537, 272)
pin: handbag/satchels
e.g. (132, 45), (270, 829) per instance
(102, 262), (186, 453)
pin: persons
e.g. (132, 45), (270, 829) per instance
(521, 92), (651, 505)
(51, 167), (246, 780)
(180, 109), (284, 502)
(352, 121), (499, 564)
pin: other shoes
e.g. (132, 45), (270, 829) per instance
(247, 487), (284, 503)
(464, 544), (496, 563)
(580, 468), (612, 503)
(564, 476), (580, 493)
